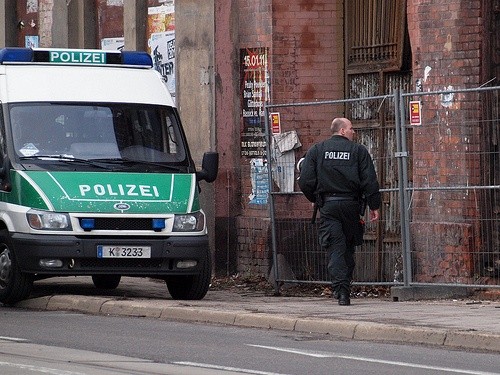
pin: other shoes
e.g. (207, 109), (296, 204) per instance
(334, 291), (350, 305)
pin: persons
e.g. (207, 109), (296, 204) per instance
(297, 117), (382, 306)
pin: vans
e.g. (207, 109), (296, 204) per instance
(0, 47), (220, 303)
